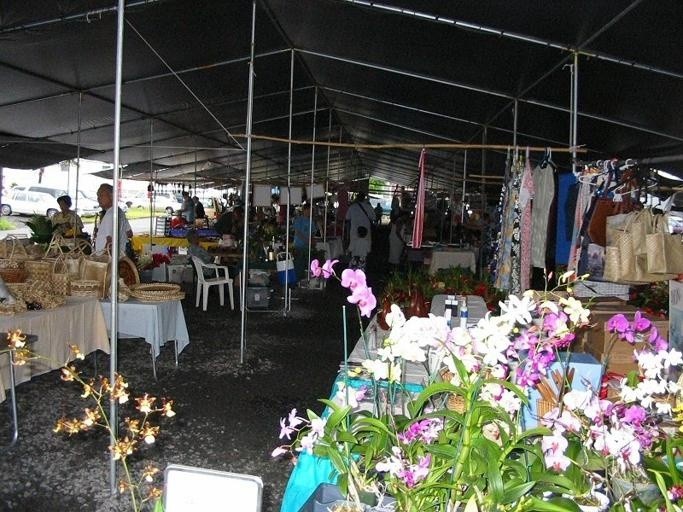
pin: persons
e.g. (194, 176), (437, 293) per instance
(293, 204), (316, 249)
(213, 207), (243, 235)
(388, 206), (409, 273)
(51, 195), (84, 236)
(346, 194), (383, 273)
(95, 184), (133, 260)
(180, 192), (205, 223)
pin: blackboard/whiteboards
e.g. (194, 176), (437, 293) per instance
(164, 464), (264, 512)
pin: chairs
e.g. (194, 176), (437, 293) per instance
(191, 254), (235, 313)
(0, 330), (39, 449)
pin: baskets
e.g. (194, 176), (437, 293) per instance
(438, 367), (467, 413)
(0, 234), (185, 315)
(537, 399), (569, 428)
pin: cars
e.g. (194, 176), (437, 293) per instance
(0, 181), (224, 220)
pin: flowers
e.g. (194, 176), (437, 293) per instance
(269, 257), (683, 512)
(152, 253), (171, 269)
(3, 327), (177, 512)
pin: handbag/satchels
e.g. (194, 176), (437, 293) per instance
(588, 196), (683, 285)
(371, 223), (376, 233)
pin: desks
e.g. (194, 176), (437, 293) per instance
(0, 296), (111, 381)
(130, 233), (220, 256)
(432, 289), (491, 331)
(98, 297), (191, 384)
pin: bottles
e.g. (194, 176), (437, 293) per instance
(267, 245), (274, 262)
(460, 292), (468, 328)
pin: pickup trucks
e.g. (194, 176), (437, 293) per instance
(370, 198), (393, 217)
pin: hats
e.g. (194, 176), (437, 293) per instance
(57, 196), (72, 207)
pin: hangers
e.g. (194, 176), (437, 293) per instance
(503, 143), (559, 176)
(573, 156), (660, 198)
